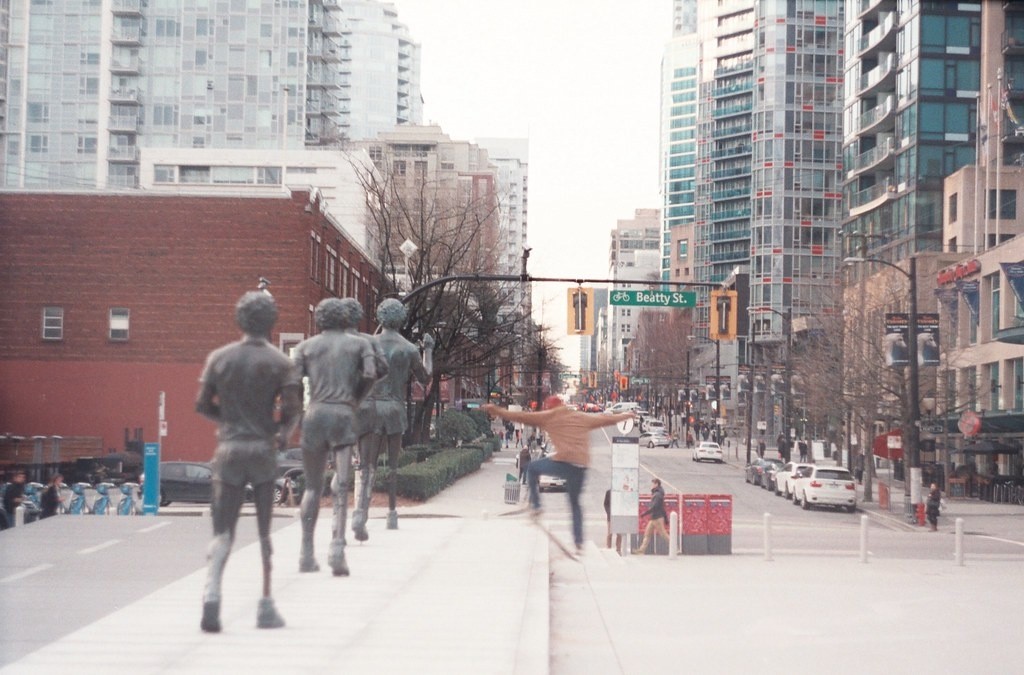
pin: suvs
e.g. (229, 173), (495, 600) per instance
(138, 459), (295, 508)
(633, 410), (666, 436)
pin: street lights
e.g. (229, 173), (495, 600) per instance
(687, 334), (721, 445)
(841, 253), (924, 521)
(745, 304), (792, 466)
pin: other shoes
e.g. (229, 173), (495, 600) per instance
(500, 444), (532, 448)
(618, 550), (622, 556)
(572, 543), (585, 555)
(630, 549), (646, 555)
(522, 482), (528, 485)
(929, 526), (937, 532)
(528, 506), (542, 516)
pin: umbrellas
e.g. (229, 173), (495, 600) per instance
(949, 440), (1018, 455)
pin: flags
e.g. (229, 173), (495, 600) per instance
(884, 312), (909, 367)
(916, 313), (941, 367)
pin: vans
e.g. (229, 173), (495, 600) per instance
(604, 401), (638, 417)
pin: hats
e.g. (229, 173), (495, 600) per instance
(545, 395), (562, 409)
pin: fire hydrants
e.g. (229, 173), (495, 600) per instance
(914, 500), (927, 527)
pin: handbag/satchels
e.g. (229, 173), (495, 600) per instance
(778, 453), (781, 460)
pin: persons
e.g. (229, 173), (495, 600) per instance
(631, 478), (671, 554)
(193, 289), (302, 633)
(798, 438), (807, 463)
(603, 489), (622, 557)
(755, 439), (765, 459)
(488, 404), (731, 454)
(370, 297), (435, 529)
(856, 451), (865, 485)
(481, 395), (637, 557)
(517, 445), (531, 484)
(291, 296), (375, 576)
(776, 434), (794, 463)
(39, 473), (67, 519)
(898, 458), (999, 532)
(337, 297), (389, 541)
(0, 469), (27, 528)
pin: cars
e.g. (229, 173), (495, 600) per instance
(566, 402), (603, 412)
(692, 439), (724, 462)
(268, 448), (308, 478)
(744, 458), (858, 512)
(637, 433), (670, 449)
